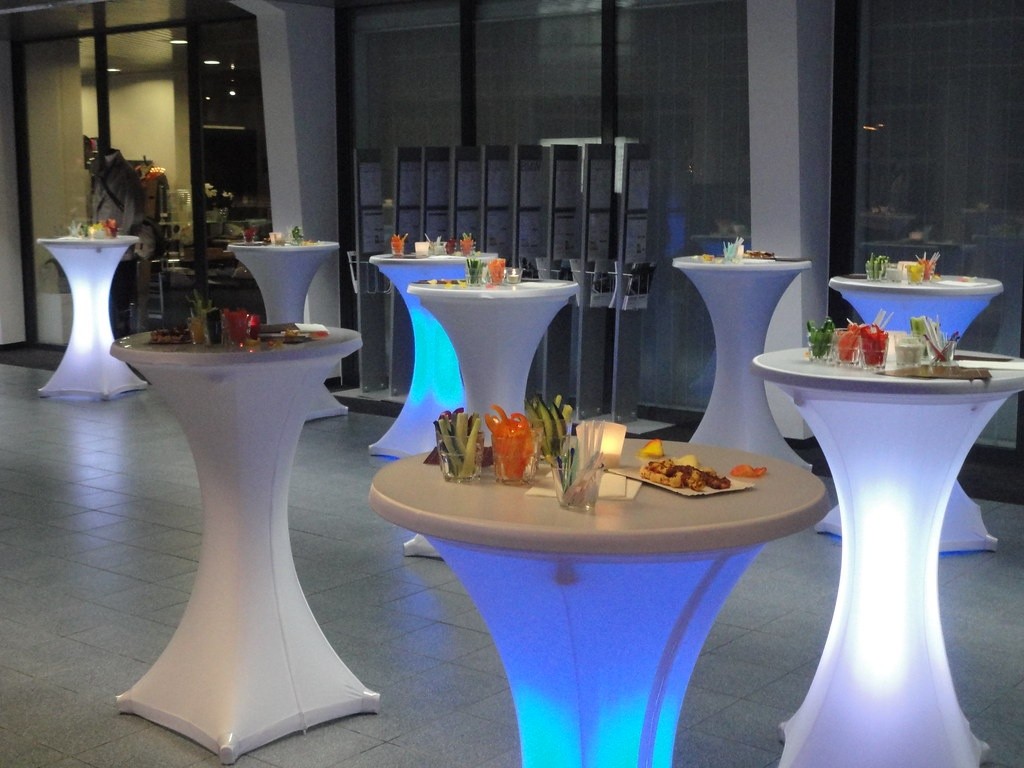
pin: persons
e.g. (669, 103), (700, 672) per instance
(91, 149), (143, 339)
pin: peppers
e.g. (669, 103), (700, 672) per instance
(487, 258), (506, 283)
(806, 316), (890, 367)
(391, 233), (408, 253)
(485, 403), (531, 480)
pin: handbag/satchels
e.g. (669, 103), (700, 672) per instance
(132, 216), (166, 259)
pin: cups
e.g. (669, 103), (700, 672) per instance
(724, 245), (744, 262)
(529, 413), (572, 464)
(415, 240), (476, 257)
(885, 331), (957, 367)
(391, 241), (404, 256)
(805, 327), (890, 370)
(491, 432), (539, 486)
(186, 302), (261, 352)
(269, 231), (302, 247)
(436, 431), (485, 483)
(465, 266), (525, 286)
(241, 229), (256, 245)
(551, 462), (604, 509)
(68, 225), (119, 239)
(528, 425), (544, 471)
(866, 260), (936, 283)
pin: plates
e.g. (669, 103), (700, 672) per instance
(607, 466), (754, 496)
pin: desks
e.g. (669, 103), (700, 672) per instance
(672, 256), (814, 472)
(109, 327), (382, 765)
(749, 348), (1024, 768)
(34, 235), (147, 403)
(805, 275), (1005, 555)
(226, 241), (350, 419)
(374, 443), (829, 768)
(403, 279), (581, 560)
(364, 252), (498, 463)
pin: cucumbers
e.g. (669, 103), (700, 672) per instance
(866, 252), (888, 280)
(466, 258), (484, 283)
(432, 408), (481, 480)
(909, 316), (929, 336)
(524, 393), (573, 460)
